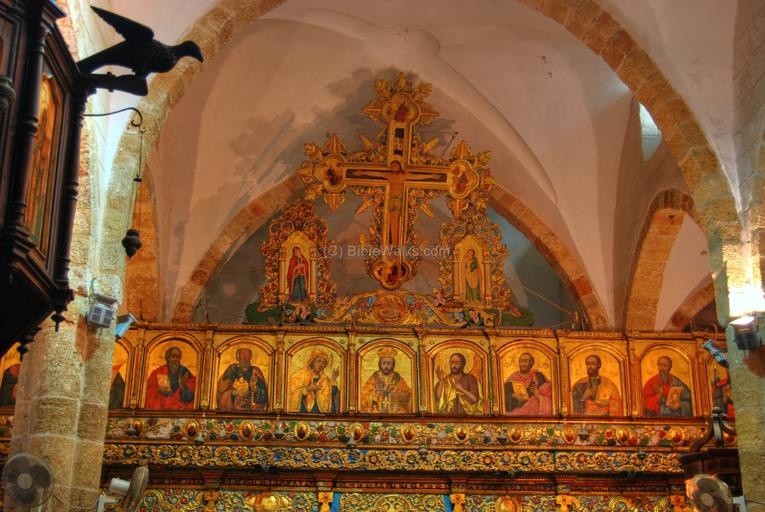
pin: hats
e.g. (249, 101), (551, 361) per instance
(307, 348), (329, 369)
(376, 347), (398, 357)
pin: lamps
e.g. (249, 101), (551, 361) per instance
(86, 292), (118, 328)
(729, 316), (759, 352)
(117, 314), (138, 338)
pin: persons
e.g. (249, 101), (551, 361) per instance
(145, 347), (195, 410)
(287, 349), (340, 414)
(504, 353), (552, 417)
(435, 353), (484, 416)
(286, 247), (312, 304)
(643, 356), (692, 417)
(572, 355), (622, 417)
(462, 249), (482, 301)
(216, 346), (268, 412)
(360, 347), (411, 413)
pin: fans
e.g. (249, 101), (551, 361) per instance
(685, 475), (733, 512)
(2, 452), (54, 512)
(96, 465), (150, 512)
